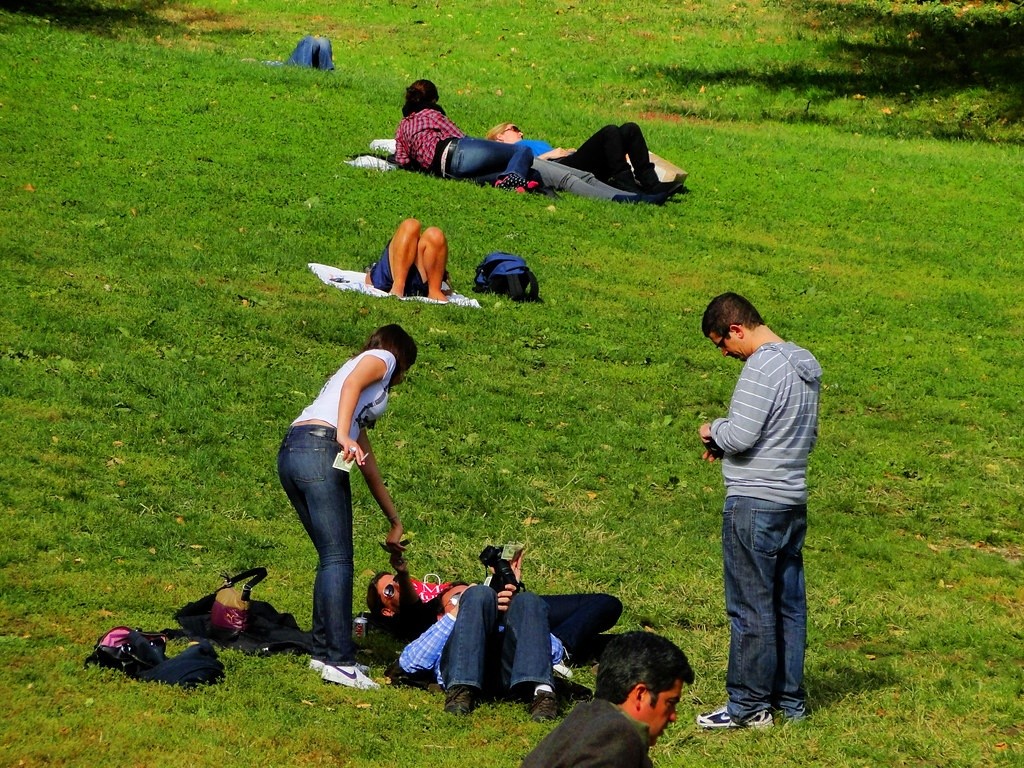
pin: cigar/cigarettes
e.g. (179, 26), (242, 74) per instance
(361, 453), (369, 462)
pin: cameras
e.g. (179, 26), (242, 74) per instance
(479, 545), (520, 595)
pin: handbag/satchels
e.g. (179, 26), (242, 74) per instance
(85, 625), (167, 675)
(210, 567), (267, 640)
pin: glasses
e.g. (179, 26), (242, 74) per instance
(502, 126), (523, 139)
(442, 593), (461, 608)
(717, 324), (741, 351)
(382, 574), (401, 605)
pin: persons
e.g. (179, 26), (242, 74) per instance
(367, 551), (623, 723)
(277, 324), (417, 691)
(241, 34), (334, 71)
(697, 291), (822, 728)
(518, 631), (695, 768)
(366, 217), (453, 302)
(394, 79), (684, 205)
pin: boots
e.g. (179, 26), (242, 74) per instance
(633, 163), (683, 200)
(608, 167), (651, 196)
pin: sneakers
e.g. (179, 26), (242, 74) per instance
(444, 684), (474, 716)
(696, 704), (774, 727)
(529, 690), (557, 722)
(309, 659), (326, 673)
(321, 664), (381, 690)
(552, 646), (572, 678)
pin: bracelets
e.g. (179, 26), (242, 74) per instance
(389, 515), (397, 521)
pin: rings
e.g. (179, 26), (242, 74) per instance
(348, 445), (356, 452)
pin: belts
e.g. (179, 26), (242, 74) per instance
(445, 139), (458, 180)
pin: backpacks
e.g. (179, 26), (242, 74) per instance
(473, 252), (538, 302)
